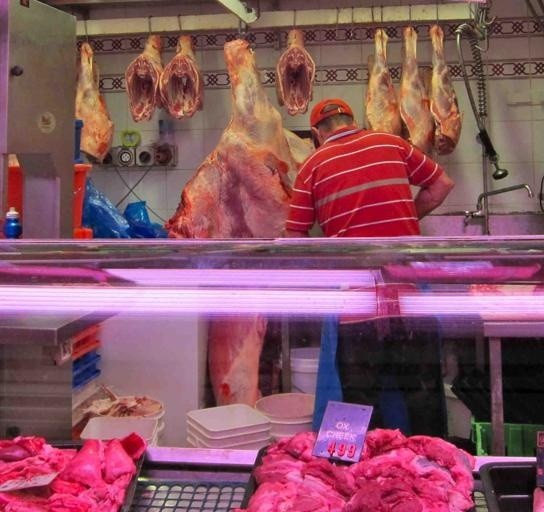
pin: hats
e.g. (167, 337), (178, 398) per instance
(310, 99), (354, 150)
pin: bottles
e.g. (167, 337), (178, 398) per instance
(2, 207), (22, 238)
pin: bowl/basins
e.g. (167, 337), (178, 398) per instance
(78, 402), (271, 450)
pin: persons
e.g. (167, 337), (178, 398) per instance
(283, 97), (457, 440)
